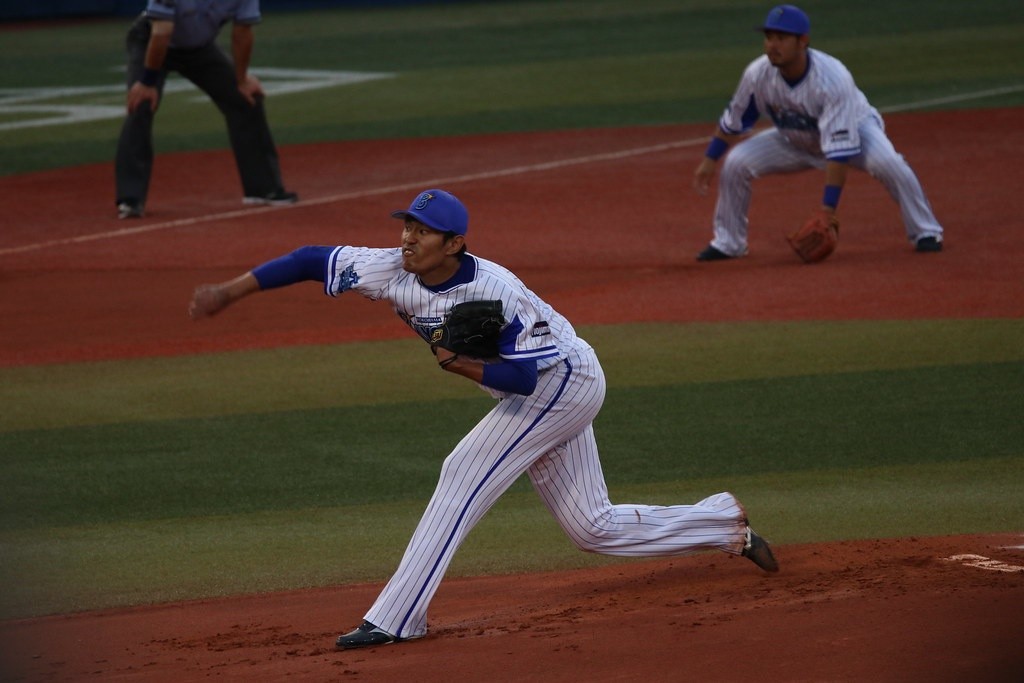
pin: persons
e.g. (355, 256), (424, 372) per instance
(696, 6), (943, 262)
(114, 0), (298, 219)
(189, 190), (778, 645)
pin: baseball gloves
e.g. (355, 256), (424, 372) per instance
(430, 299), (507, 370)
(783, 210), (840, 266)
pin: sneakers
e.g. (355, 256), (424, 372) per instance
(335, 621), (406, 648)
(742, 521), (777, 572)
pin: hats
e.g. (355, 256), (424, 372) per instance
(754, 4), (810, 37)
(391, 189), (469, 235)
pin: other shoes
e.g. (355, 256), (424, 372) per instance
(696, 246), (726, 260)
(118, 201), (144, 219)
(915, 235), (942, 252)
(243, 190), (297, 204)
